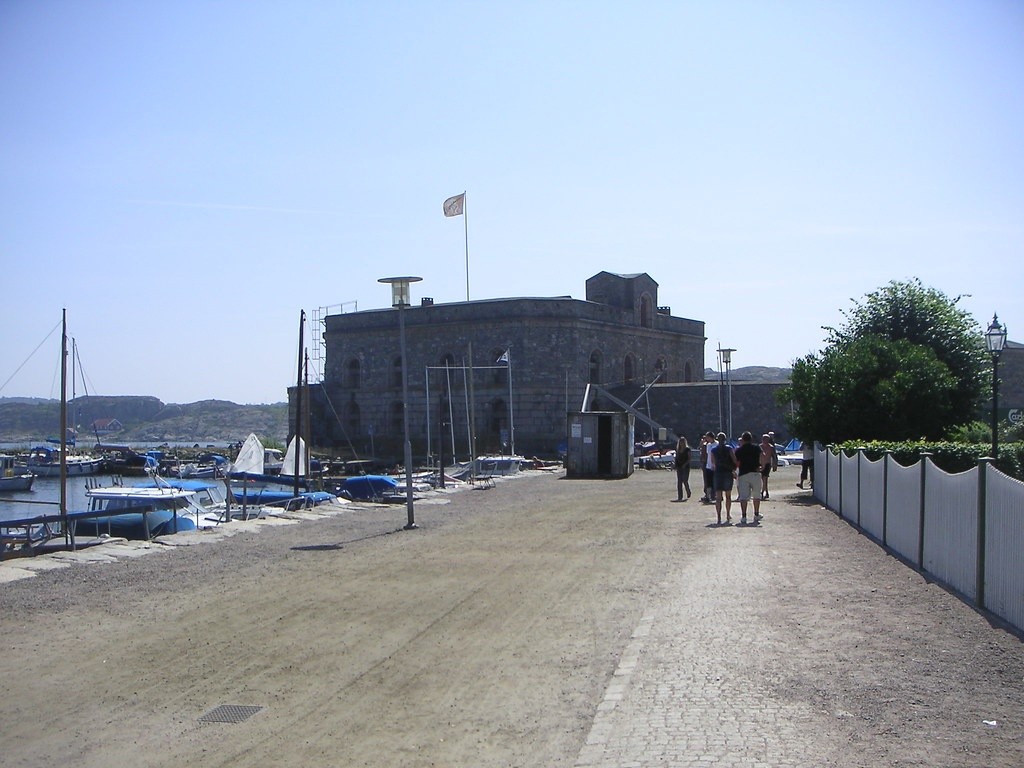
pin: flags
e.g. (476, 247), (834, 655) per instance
(443, 193), (464, 217)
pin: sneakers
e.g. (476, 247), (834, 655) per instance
(754, 513), (763, 522)
(741, 517), (747, 524)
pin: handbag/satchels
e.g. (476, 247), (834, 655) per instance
(731, 469), (737, 479)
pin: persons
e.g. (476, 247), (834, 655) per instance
(735, 431), (766, 523)
(699, 434), (707, 501)
(796, 441), (814, 489)
(768, 432), (775, 447)
(675, 437), (691, 501)
(711, 433), (740, 526)
(759, 434), (778, 500)
(705, 431), (719, 504)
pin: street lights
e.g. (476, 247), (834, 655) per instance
(377, 276), (425, 530)
(983, 311), (1009, 459)
(716, 348), (738, 444)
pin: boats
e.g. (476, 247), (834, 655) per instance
(633, 453), (675, 465)
(176, 456), (234, 478)
(65, 473), (465, 539)
(217, 449), (285, 475)
(104, 451), (159, 475)
(687, 341), (805, 467)
(0, 453), (38, 491)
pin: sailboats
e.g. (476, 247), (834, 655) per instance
(259, 306), (340, 511)
(303, 346), (356, 505)
(0, 307), (127, 563)
(28, 336), (105, 477)
(466, 348), (524, 478)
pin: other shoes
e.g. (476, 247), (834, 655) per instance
(677, 496), (683, 502)
(699, 496), (723, 505)
(797, 484), (803, 489)
(687, 491), (691, 498)
(718, 519), (721, 524)
(760, 492), (769, 500)
(727, 516), (732, 520)
